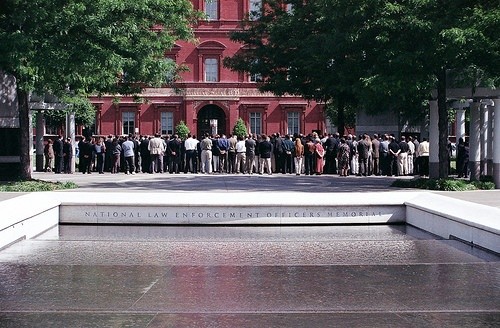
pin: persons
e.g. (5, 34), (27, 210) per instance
(11, 123), (476, 179)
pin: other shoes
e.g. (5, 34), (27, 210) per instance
(43, 166), (429, 176)
